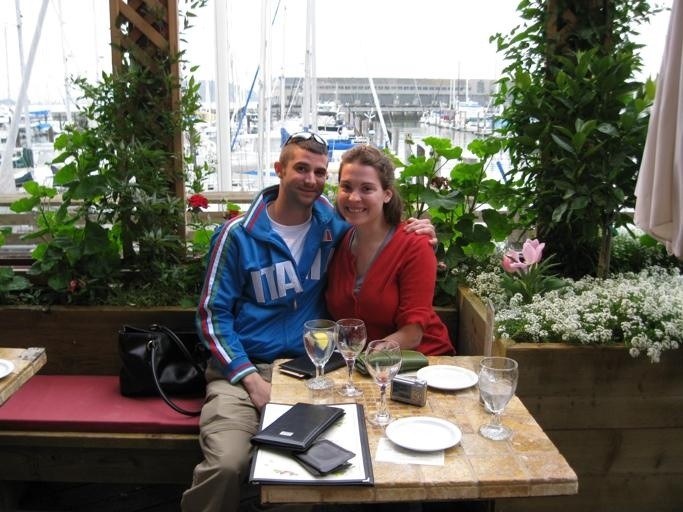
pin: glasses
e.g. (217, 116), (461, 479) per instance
(283, 131), (328, 147)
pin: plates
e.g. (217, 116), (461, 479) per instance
(0, 359), (15, 380)
(417, 364), (479, 392)
(386, 416), (462, 452)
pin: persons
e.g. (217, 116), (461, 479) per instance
(325, 144), (458, 359)
(180, 131), (439, 512)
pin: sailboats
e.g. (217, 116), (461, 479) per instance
(419, 78), (520, 140)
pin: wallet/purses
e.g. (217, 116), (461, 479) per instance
(279, 350), (346, 378)
(250, 402), (345, 453)
(293, 440), (355, 476)
(354, 350), (429, 376)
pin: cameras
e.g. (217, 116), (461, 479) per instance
(389, 374), (428, 408)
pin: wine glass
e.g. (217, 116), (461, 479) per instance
(477, 356), (519, 442)
(303, 319), (338, 390)
(334, 319), (367, 398)
(363, 340), (402, 428)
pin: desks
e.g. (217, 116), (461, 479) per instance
(258, 353), (580, 511)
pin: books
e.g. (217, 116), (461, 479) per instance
(249, 402), (375, 487)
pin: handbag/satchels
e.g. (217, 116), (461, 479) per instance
(117, 323), (207, 397)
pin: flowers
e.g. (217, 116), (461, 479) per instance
(186, 192), (239, 245)
(467, 235), (683, 364)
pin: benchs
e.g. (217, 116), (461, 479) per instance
(0, 306), (200, 487)
